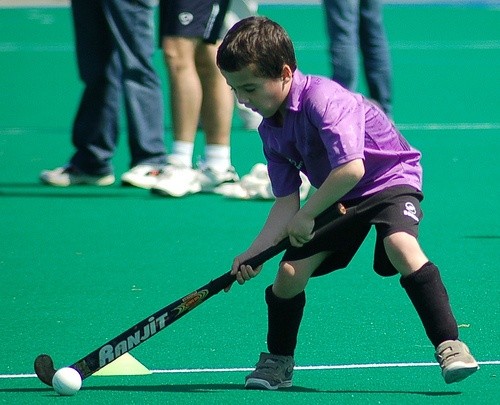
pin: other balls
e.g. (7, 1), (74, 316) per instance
(52, 367), (83, 396)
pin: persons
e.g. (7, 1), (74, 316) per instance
(217, 17), (479, 391)
(322, 0), (392, 120)
(197, 0), (266, 131)
(151, 1), (239, 198)
(39, 0), (169, 191)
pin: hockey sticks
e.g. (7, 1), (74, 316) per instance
(35, 201), (348, 388)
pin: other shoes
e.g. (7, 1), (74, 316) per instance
(194, 161), (240, 193)
(150, 162), (202, 197)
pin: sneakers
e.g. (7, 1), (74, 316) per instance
(245, 352), (295, 390)
(121, 161), (161, 190)
(434, 339), (480, 384)
(40, 162), (115, 186)
